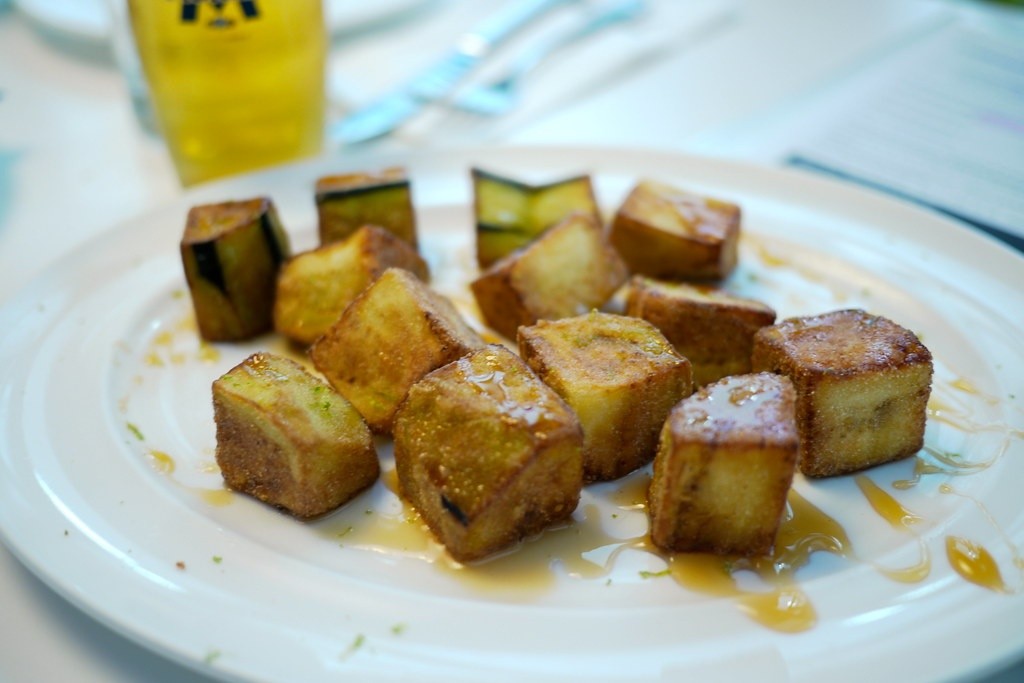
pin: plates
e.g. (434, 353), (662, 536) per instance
(1, 144), (1024, 683)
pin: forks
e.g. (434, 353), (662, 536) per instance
(419, 2), (649, 115)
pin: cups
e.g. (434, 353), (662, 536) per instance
(125, 1), (326, 190)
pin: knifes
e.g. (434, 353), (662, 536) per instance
(322, 1), (550, 143)
